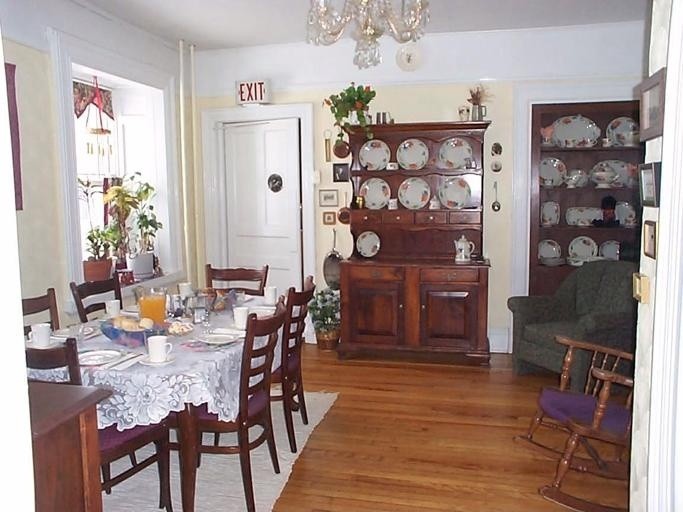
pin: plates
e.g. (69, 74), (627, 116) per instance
(337, 208), (352, 224)
(354, 136), (476, 212)
(355, 230), (380, 258)
(537, 112), (640, 269)
(333, 139), (350, 158)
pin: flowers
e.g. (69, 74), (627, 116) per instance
(308, 288), (340, 332)
(321, 81), (376, 140)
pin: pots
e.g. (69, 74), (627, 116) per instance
(321, 228), (345, 291)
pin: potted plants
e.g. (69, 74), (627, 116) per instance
(103, 171), (163, 281)
(75, 176), (112, 282)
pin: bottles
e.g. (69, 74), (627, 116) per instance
(381, 111), (385, 125)
(376, 111), (381, 126)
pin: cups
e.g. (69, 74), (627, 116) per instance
(25, 282), (280, 371)
(115, 259), (135, 285)
(457, 105), (470, 122)
(470, 104), (486, 122)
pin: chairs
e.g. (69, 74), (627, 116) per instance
(21, 264), (315, 512)
(512, 335), (634, 512)
(507, 260), (641, 396)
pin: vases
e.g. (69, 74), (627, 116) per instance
(315, 328), (337, 350)
(348, 110), (368, 125)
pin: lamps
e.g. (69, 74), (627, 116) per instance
(306, 0), (429, 69)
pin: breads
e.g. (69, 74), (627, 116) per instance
(113, 316), (153, 332)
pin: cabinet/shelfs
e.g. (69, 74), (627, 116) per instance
(333, 120), (492, 368)
(528, 100), (644, 297)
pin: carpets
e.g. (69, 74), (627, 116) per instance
(98, 389), (340, 512)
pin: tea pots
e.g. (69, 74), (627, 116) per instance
(452, 233), (474, 263)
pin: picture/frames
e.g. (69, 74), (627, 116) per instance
(323, 212), (336, 225)
(638, 66), (666, 142)
(643, 220), (656, 259)
(637, 161), (660, 207)
(333, 163), (349, 182)
(318, 189), (338, 207)
